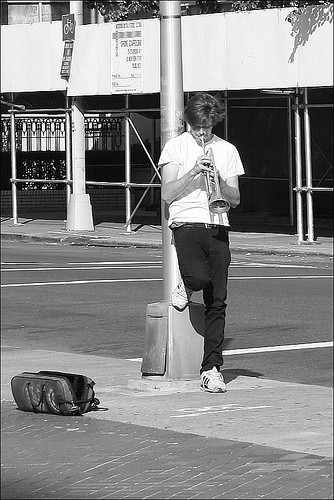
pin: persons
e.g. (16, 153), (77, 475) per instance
(158, 92), (245, 392)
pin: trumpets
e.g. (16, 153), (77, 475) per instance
(200, 131), (230, 214)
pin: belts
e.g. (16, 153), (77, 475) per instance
(183, 223), (231, 231)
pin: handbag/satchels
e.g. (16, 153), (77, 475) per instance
(10, 370), (109, 416)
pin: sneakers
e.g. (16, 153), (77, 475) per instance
(171, 279), (194, 312)
(199, 365), (227, 393)
